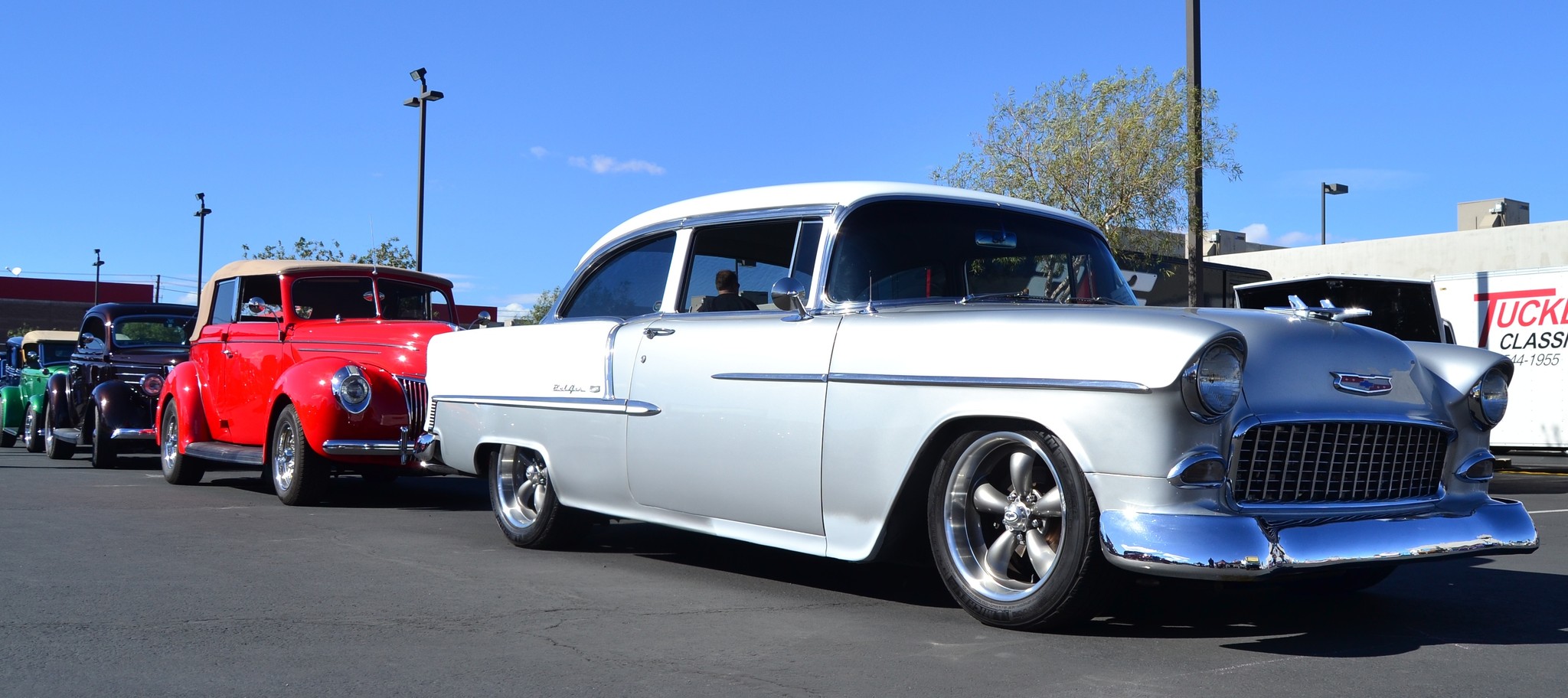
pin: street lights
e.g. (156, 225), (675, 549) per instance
(92, 249), (104, 306)
(1321, 181), (1348, 244)
(406, 64), (444, 271)
(194, 192), (212, 317)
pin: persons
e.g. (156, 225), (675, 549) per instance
(697, 270), (761, 314)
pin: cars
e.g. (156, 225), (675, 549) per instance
(45, 302), (199, 469)
(155, 259), (492, 504)
(414, 180), (1540, 632)
(0, 330), (132, 453)
